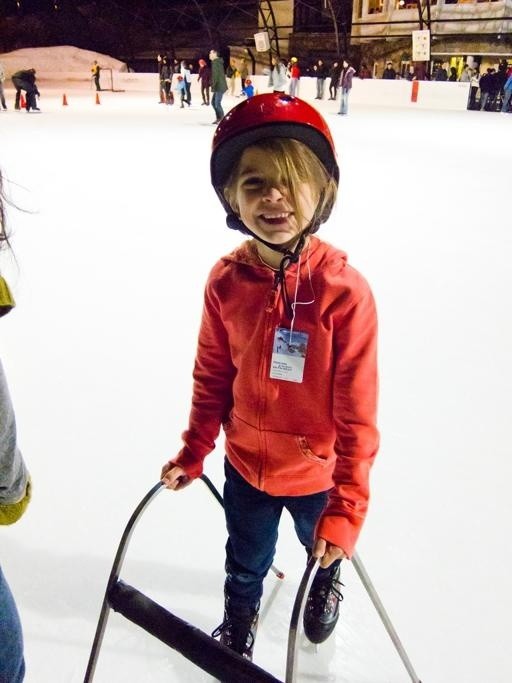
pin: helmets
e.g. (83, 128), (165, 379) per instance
(210, 93), (339, 224)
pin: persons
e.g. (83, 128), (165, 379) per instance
(467, 65), (512, 113)
(359, 60), (471, 82)
(90, 60), (102, 90)
(157, 55), (191, 108)
(11, 67), (37, 110)
(0, 70), (8, 110)
(271, 56), (300, 94)
(209, 49), (228, 124)
(226, 59), (254, 98)
(198, 59), (211, 106)
(160, 95), (378, 659)
(314, 60), (356, 114)
(0, 170), (40, 682)
(26, 83), (41, 111)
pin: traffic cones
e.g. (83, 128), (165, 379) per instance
(61, 92), (68, 105)
(19, 93), (28, 108)
(253, 88), (262, 97)
(94, 92), (101, 104)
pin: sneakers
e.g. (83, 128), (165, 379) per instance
(212, 607), (257, 657)
(303, 547), (346, 644)
